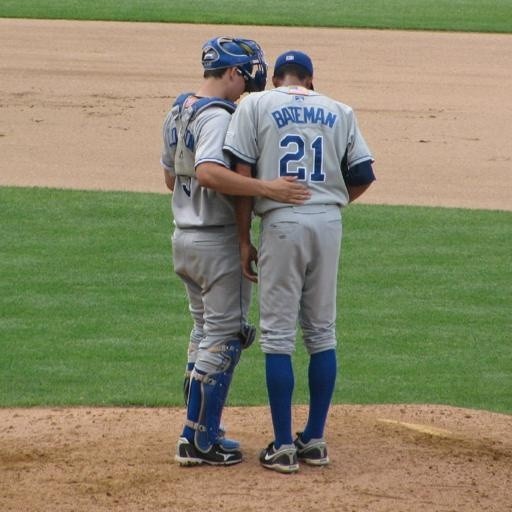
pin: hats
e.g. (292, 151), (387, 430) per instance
(274, 50), (315, 91)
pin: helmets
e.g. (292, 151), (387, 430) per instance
(201, 35), (268, 92)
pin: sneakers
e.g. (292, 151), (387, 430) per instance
(259, 431), (330, 474)
(174, 432), (244, 467)
